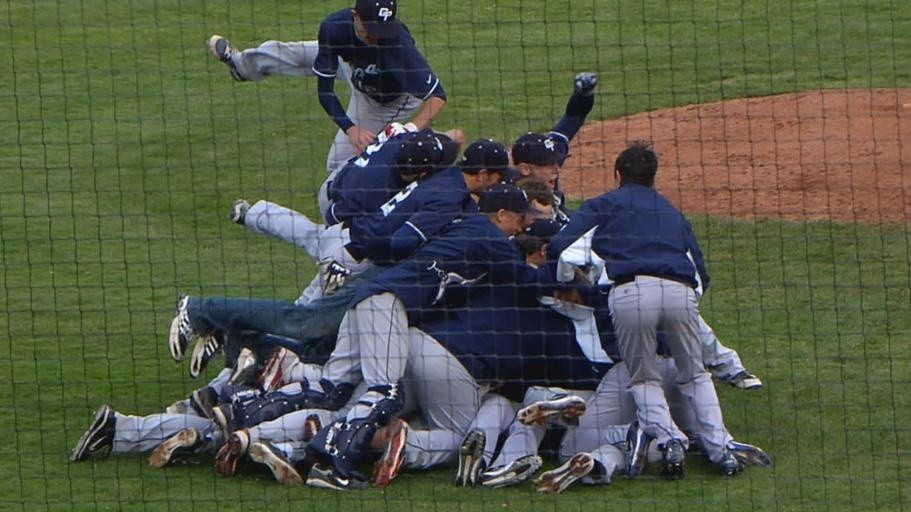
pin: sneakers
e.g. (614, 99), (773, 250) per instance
(228, 198), (249, 224)
(317, 255), (354, 300)
(247, 439), (303, 486)
(189, 328), (226, 379)
(627, 421), (651, 479)
(516, 395), (586, 427)
(147, 427), (203, 468)
(166, 345), (302, 476)
(724, 440), (772, 469)
(728, 367), (762, 390)
(535, 452), (594, 493)
(479, 454), (543, 491)
(666, 440), (687, 480)
(373, 419), (410, 488)
(71, 403), (121, 463)
(305, 464), (369, 492)
(168, 292), (195, 361)
(209, 34), (250, 82)
(304, 411), (323, 442)
(454, 428), (486, 490)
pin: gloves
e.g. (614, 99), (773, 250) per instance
(574, 71), (597, 95)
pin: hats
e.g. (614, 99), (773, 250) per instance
(458, 138), (509, 168)
(512, 131), (572, 165)
(356, 0), (399, 40)
(477, 184), (542, 215)
(396, 133), (457, 173)
(525, 219), (562, 238)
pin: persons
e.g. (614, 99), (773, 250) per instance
(76, 125), (773, 492)
(511, 69), (600, 208)
(207, 2), (448, 176)
